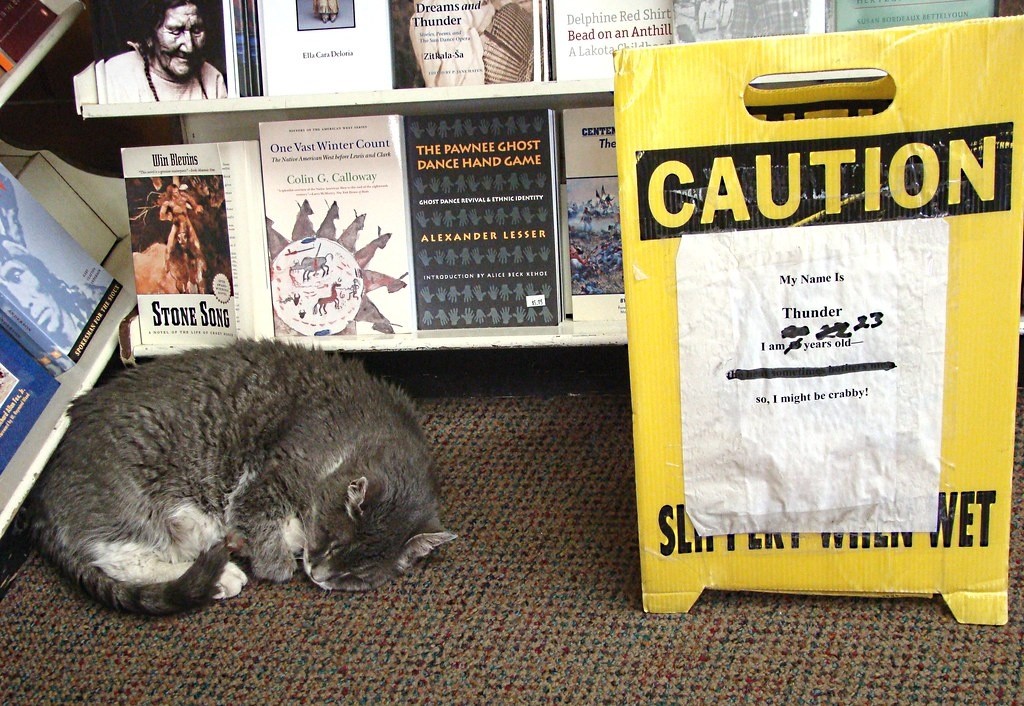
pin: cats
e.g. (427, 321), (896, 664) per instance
(36, 335), (461, 618)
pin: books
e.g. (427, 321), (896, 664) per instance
(0, 0), (1024, 488)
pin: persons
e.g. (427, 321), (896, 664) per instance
(103, 0), (227, 105)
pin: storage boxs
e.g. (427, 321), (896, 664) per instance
(612, 13), (1024, 628)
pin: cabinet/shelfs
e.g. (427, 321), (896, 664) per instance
(0, 0), (138, 544)
(81, 77), (628, 362)
